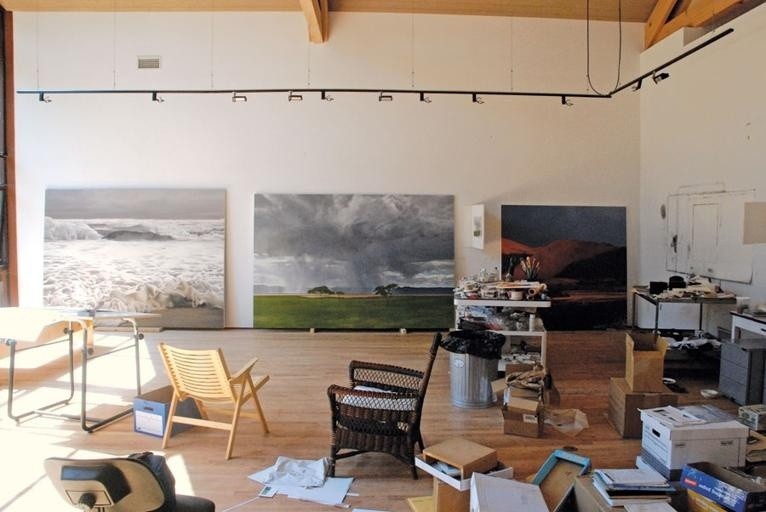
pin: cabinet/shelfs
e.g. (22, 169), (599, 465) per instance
(450, 299), (551, 372)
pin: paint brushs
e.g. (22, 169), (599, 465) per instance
(519, 255), (542, 280)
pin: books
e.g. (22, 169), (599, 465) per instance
(591, 468), (677, 505)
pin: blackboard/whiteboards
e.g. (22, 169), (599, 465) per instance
(665, 188), (759, 283)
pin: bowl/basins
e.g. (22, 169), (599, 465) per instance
(700, 390), (719, 399)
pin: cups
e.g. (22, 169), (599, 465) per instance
(510, 292), (523, 298)
(736, 296), (751, 314)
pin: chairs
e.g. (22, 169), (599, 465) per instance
(157, 343), (270, 461)
(327, 332), (442, 480)
(44, 453), (216, 511)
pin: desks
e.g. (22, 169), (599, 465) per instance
(632, 290), (737, 342)
(0, 306), (162, 434)
(730, 311), (766, 344)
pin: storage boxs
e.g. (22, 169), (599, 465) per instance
(133, 384), (202, 439)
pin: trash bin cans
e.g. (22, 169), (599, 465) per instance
(441, 329), (505, 409)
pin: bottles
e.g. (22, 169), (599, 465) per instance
(529, 314), (536, 330)
(478, 267), (499, 283)
